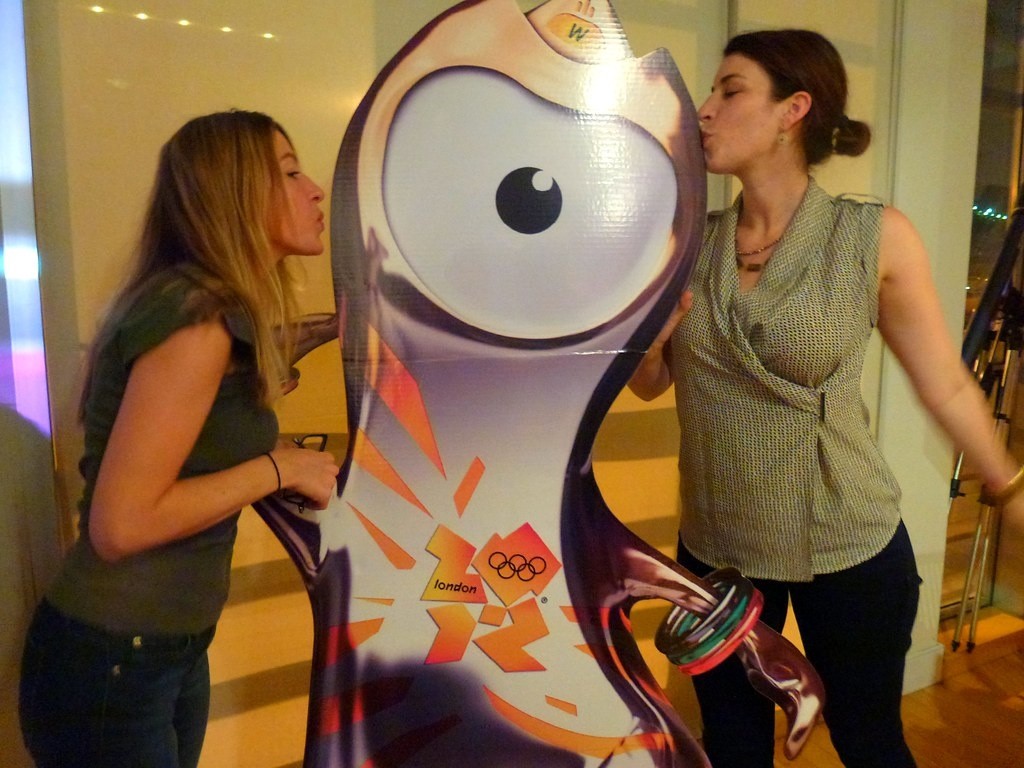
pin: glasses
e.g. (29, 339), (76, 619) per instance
(280, 432), (327, 515)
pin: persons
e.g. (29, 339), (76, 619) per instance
(628, 29), (1024, 768)
(15, 112), (339, 768)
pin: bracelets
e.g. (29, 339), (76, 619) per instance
(653, 567), (764, 678)
(266, 454), (282, 493)
(981, 470), (1024, 508)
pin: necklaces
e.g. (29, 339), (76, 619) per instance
(733, 221), (783, 271)
